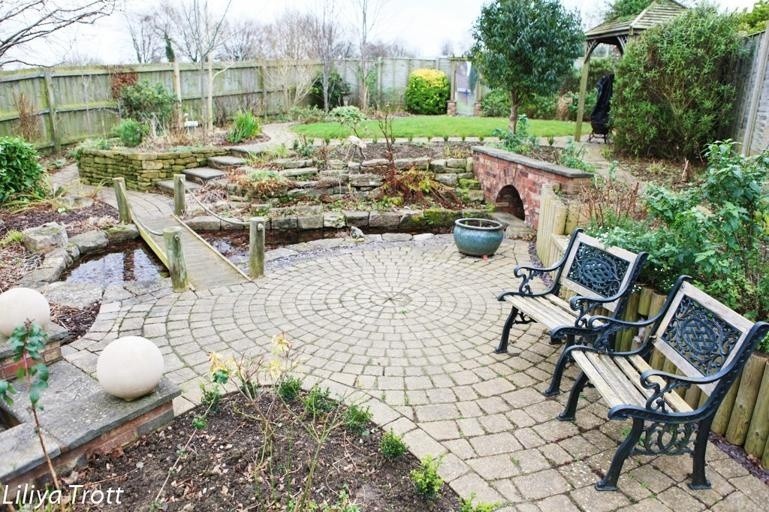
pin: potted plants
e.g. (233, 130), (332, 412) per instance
(453, 216), (505, 259)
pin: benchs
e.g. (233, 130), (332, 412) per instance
(494, 227), (650, 398)
(554, 274), (769, 492)
